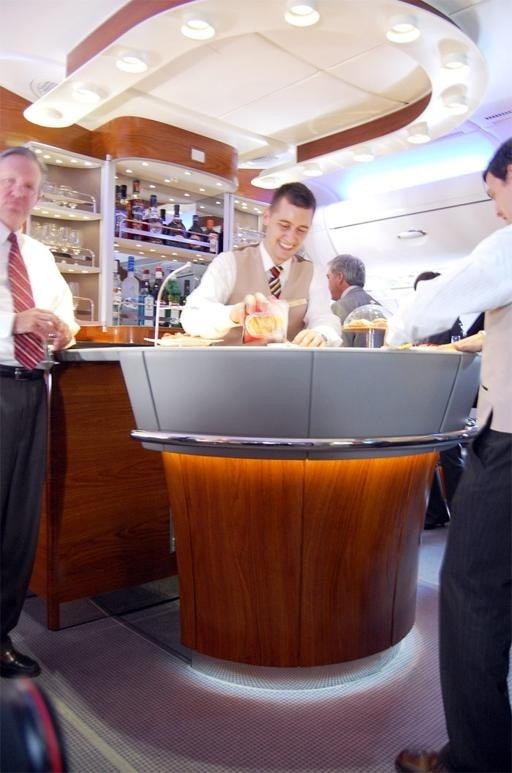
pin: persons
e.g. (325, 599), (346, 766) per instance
(383, 137), (512, 773)
(414, 272), (465, 529)
(0, 146), (82, 679)
(179, 182), (343, 349)
(327, 254), (386, 346)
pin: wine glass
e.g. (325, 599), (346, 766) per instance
(41, 181), (79, 207)
(38, 331), (59, 364)
(69, 281), (80, 320)
(30, 221), (81, 267)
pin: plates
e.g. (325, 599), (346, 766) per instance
(268, 343), (305, 348)
(144, 337), (223, 345)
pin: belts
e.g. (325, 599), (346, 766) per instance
(0, 367), (45, 380)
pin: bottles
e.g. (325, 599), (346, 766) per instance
(204, 220), (220, 253)
(139, 269), (151, 326)
(122, 256), (137, 326)
(187, 216), (204, 251)
(116, 184), (120, 208)
(146, 194), (163, 244)
(181, 280), (191, 325)
(168, 205), (187, 246)
(131, 180), (144, 240)
(220, 222), (223, 253)
(114, 259), (121, 296)
(153, 267), (168, 327)
(159, 208), (167, 244)
(117, 186), (131, 237)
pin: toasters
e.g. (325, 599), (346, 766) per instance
(167, 269), (181, 326)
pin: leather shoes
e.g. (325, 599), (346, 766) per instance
(395, 749), (451, 773)
(0, 648), (40, 678)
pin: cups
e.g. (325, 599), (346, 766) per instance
(244, 300), (288, 342)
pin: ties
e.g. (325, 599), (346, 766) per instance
(8, 233), (44, 371)
(269, 266), (281, 298)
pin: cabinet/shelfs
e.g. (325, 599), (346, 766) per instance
(232, 193), (271, 250)
(22, 139), (110, 327)
(113, 157), (237, 327)
